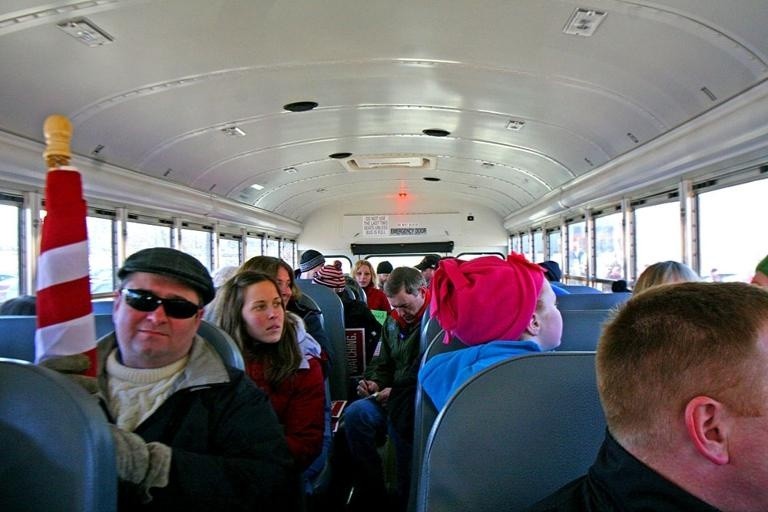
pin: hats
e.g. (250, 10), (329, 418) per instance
(416, 255), (442, 270)
(312, 262), (346, 288)
(299, 250), (323, 272)
(428, 252), (546, 345)
(378, 262), (392, 275)
(117, 248), (216, 304)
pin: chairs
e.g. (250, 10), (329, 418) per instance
(419, 291), (636, 358)
(0, 356), (117, 512)
(295, 282), (349, 397)
(90, 299), (117, 315)
(562, 286), (602, 294)
(415, 349), (611, 512)
(413, 308), (618, 507)
(214, 286), (325, 329)
(1, 313), (248, 369)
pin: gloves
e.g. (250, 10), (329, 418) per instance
(115, 423), (164, 504)
(40, 357), (100, 407)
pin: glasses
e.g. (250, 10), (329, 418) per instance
(121, 287), (207, 319)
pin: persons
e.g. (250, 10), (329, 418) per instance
(351, 260), (391, 327)
(294, 269), (302, 278)
(633, 261), (702, 296)
(216, 270), (324, 465)
(295, 250), (325, 281)
(612, 280), (631, 294)
(750, 255), (768, 287)
(238, 256), (334, 379)
(344, 267), (431, 512)
(528, 281), (768, 512)
(414, 254), (441, 281)
(417, 255), (563, 412)
(374, 261), (393, 290)
(34, 247), (300, 512)
(311, 260), (383, 366)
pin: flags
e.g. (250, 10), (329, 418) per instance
(34, 170), (97, 379)
